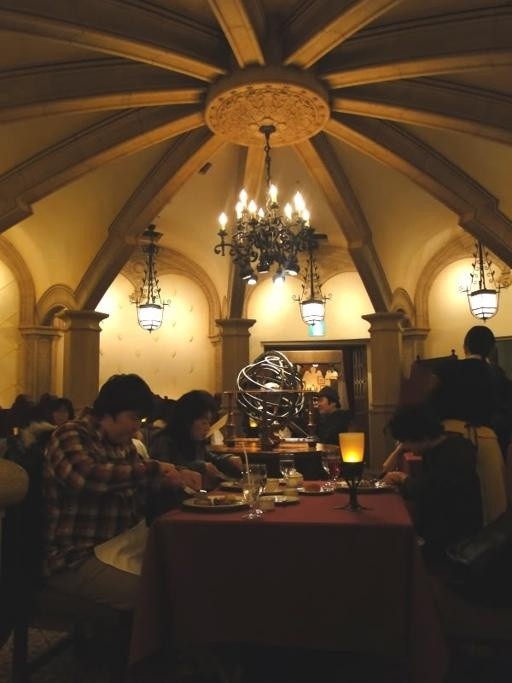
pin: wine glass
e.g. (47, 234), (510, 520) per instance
(241, 463), (268, 518)
(322, 448), (341, 491)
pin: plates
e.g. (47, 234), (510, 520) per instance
(183, 496), (247, 509)
(219, 477), (393, 505)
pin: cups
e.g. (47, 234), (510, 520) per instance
(280, 452), (296, 475)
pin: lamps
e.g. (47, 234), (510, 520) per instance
(293, 233), (328, 326)
(462, 239), (501, 322)
(215, 127), (315, 285)
(309, 320), (324, 336)
(131, 225), (168, 332)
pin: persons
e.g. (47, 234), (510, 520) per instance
(311, 385), (352, 446)
(1, 372), (248, 682)
(300, 366), (325, 393)
(375, 324), (512, 683)
(325, 366), (339, 388)
